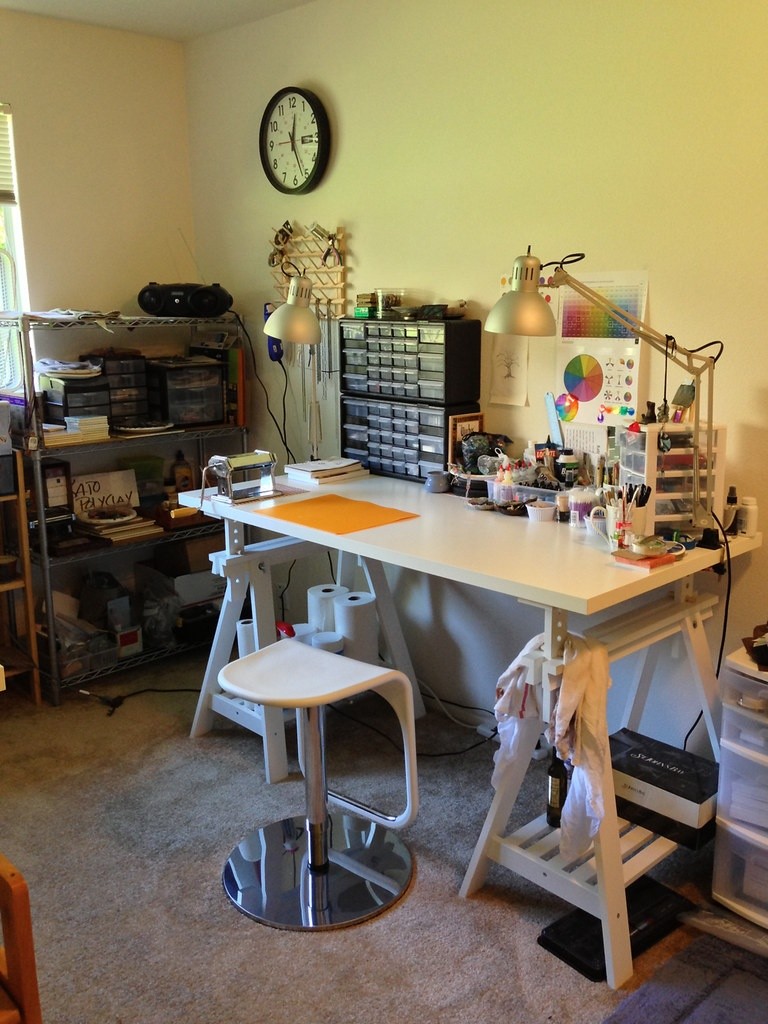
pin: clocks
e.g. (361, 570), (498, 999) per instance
(258, 87), (332, 196)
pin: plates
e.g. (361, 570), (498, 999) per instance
(467, 498), (496, 509)
(41, 367), (102, 379)
(497, 503), (526, 516)
(113, 420), (174, 433)
(76, 508), (137, 525)
(444, 315), (465, 319)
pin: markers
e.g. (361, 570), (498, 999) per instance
(605, 483), (651, 507)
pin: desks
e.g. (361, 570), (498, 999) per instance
(178, 471), (762, 991)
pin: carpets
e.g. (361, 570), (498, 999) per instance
(602, 932), (768, 1024)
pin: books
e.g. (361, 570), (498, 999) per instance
(73, 515), (164, 542)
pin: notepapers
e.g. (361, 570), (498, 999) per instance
(616, 553), (674, 568)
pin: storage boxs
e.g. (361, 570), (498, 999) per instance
(135, 560), (227, 607)
(608, 726), (719, 852)
(151, 532), (225, 578)
(484, 478), (582, 504)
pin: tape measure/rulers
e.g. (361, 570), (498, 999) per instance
(544, 392), (563, 449)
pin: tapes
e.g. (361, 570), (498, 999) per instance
(631, 537), (666, 555)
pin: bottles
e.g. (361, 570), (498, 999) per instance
(723, 486), (739, 539)
(494, 434), (555, 484)
(557, 493), (570, 522)
(546, 746), (567, 828)
(740, 496), (758, 536)
(159, 486), (178, 511)
(556, 449), (580, 488)
(613, 521), (634, 549)
(172, 450), (195, 492)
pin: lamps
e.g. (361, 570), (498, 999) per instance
(485, 244), (725, 537)
(262, 261), (322, 462)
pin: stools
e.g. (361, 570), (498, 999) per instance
(217, 637), (419, 933)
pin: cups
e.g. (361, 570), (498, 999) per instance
(595, 482), (619, 507)
(583, 514), (607, 535)
(425, 471), (451, 492)
(525, 502), (556, 521)
(590, 502), (647, 547)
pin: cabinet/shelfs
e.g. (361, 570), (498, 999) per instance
(0, 315), (250, 707)
(336, 318), (482, 484)
(711, 646), (768, 930)
(619, 419), (726, 537)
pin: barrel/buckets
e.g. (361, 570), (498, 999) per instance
(374, 288), (404, 320)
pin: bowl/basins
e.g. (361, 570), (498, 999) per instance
(391, 304), (448, 320)
